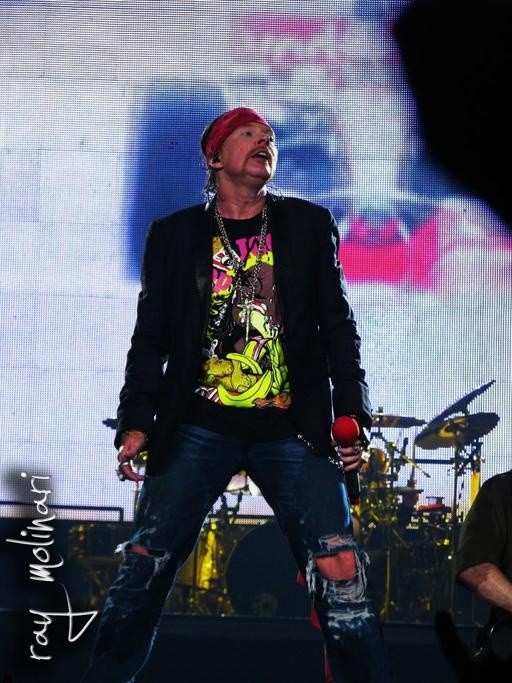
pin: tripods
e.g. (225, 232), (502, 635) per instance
(365, 430), (484, 626)
(178, 529), (214, 618)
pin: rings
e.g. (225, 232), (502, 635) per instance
(350, 444), (363, 454)
(120, 460), (130, 467)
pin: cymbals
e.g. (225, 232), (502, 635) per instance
(415, 412), (500, 449)
(370, 414), (426, 428)
(414, 378), (496, 443)
(224, 468), (262, 496)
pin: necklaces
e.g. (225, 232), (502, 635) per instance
(213, 197), (268, 344)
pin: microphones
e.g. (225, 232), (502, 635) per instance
(332, 416), (360, 506)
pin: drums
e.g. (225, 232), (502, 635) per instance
(224, 517), (312, 615)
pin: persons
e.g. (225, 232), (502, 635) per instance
(444, 465), (512, 682)
(85, 107), (393, 683)
